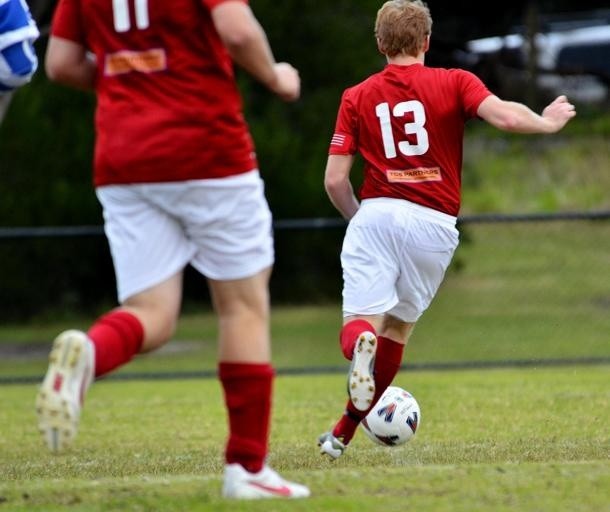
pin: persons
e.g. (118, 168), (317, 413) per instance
(317, 1), (579, 466)
(33, 0), (312, 502)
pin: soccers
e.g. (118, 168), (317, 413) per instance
(360, 386), (420, 446)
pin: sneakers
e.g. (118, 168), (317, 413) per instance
(34, 328), (96, 456)
(316, 428), (346, 462)
(217, 460), (313, 505)
(346, 329), (378, 413)
